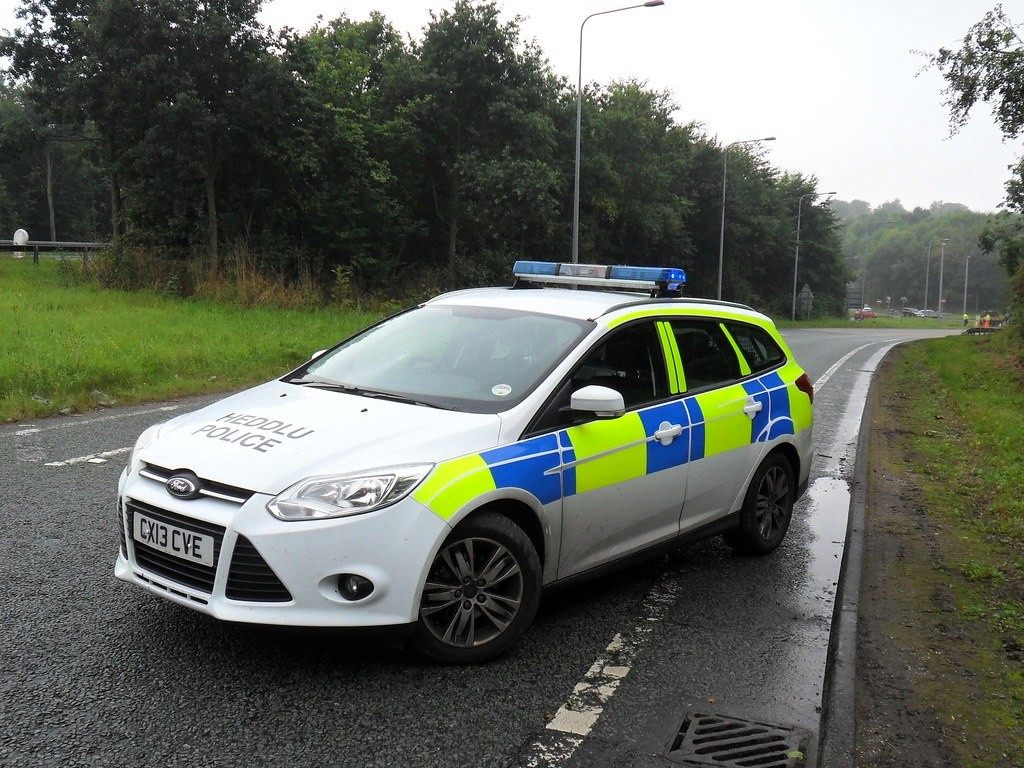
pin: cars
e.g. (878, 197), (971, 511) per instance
(854, 307), (879, 320)
(113, 261), (813, 666)
(901, 307), (937, 318)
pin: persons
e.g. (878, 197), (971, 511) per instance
(983, 313), (990, 328)
(975, 313), (981, 321)
(963, 313), (969, 326)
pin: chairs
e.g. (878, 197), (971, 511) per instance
(590, 334), (658, 401)
(675, 332), (727, 386)
(477, 316), (546, 400)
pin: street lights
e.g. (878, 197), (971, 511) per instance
(791, 192), (837, 321)
(859, 220), (895, 319)
(963, 255), (972, 313)
(569, 0), (667, 290)
(937, 242), (947, 313)
(715, 136), (777, 301)
(924, 238), (950, 309)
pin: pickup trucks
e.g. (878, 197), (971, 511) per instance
(980, 310), (1004, 324)
